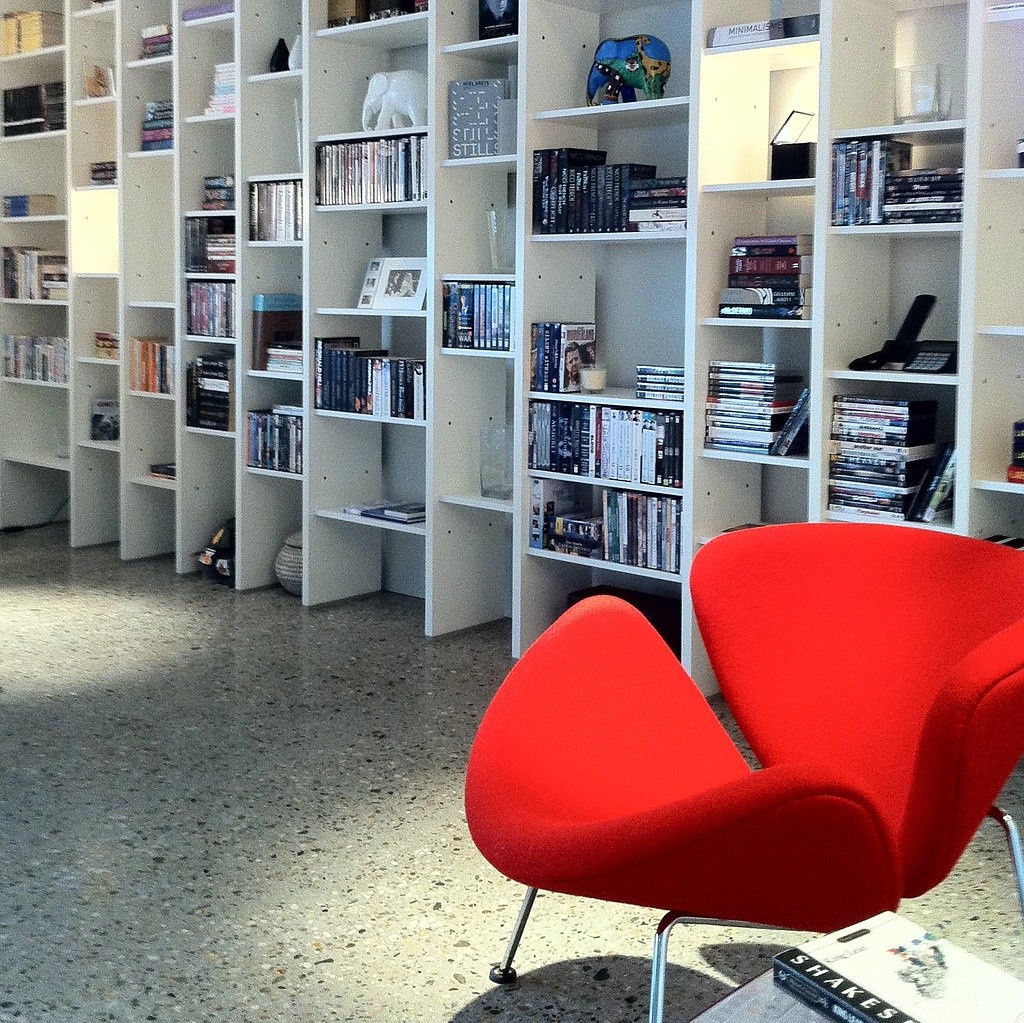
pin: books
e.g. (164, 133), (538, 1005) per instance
(440, 279), (516, 353)
(313, 334), (426, 525)
(447, 79), (510, 159)
(507, 63), (518, 100)
(478, 0), (518, 41)
(96, 338), (119, 348)
(89, 398), (120, 441)
(244, 130), (305, 478)
(0, 6), (68, 386)
(135, 22), (238, 481)
(832, 135), (963, 226)
(90, 177), (119, 186)
(90, 170), (118, 179)
(94, 345), (120, 361)
(181, 1), (234, 23)
(91, 161), (117, 171)
(326, 0), (428, 28)
(825, 394), (961, 526)
(94, 332), (120, 340)
(704, 234), (814, 460)
(314, 136), (428, 206)
(528, 147), (687, 574)
(497, 99), (518, 156)
(983, 532), (1023, 551)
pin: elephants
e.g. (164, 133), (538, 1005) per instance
(362, 69), (428, 133)
(584, 34), (670, 107)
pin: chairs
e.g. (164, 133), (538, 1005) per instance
(470, 524), (1021, 1022)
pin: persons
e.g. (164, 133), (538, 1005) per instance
(398, 272), (415, 297)
(386, 271), (401, 296)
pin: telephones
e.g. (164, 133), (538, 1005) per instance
(852, 292), (958, 372)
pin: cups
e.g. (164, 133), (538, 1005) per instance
(54, 418), (71, 458)
(576, 363), (607, 396)
(894, 62), (955, 122)
(479, 422), (514, 500)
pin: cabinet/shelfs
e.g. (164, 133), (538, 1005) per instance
(0, 1), (1022, 693)
